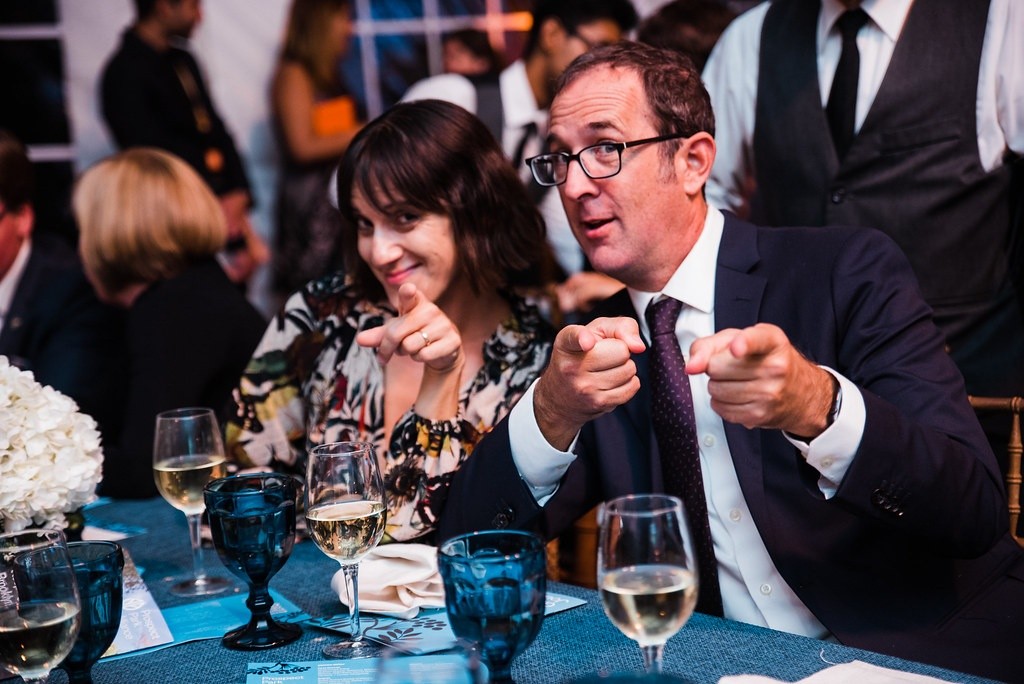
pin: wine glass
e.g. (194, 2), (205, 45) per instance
(438, 530), (547, 684)
(0, 529), (81, 684)
(598, 494), (699, 675)
(205, 473), (305, 650)
(153, 408), (230, 597)
(14, 541), (124, 684)
(305, 441), (387, 660)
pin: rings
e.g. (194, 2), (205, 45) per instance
(417, 328), (431, 345)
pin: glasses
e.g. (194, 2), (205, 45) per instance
(525, 131), (689, 187)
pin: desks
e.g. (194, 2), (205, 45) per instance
(0, 497), (1006, 684)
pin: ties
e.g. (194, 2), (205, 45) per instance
(644, 294), (724, 618)
(823, 6), (872, 164)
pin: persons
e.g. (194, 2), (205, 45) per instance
(637, 2), (734, 65)
(71, 148), (270, 501)
(435, 23), (503, 77)
(330, 0), (637, 279)
(1, 135), (89, 407)
(99, 0), (260, 298)
(228, 99), (573, 529)
(700, 0), (1023, 493)
(267, 0), (371, 281)
(440, 39), (1024, 680)
